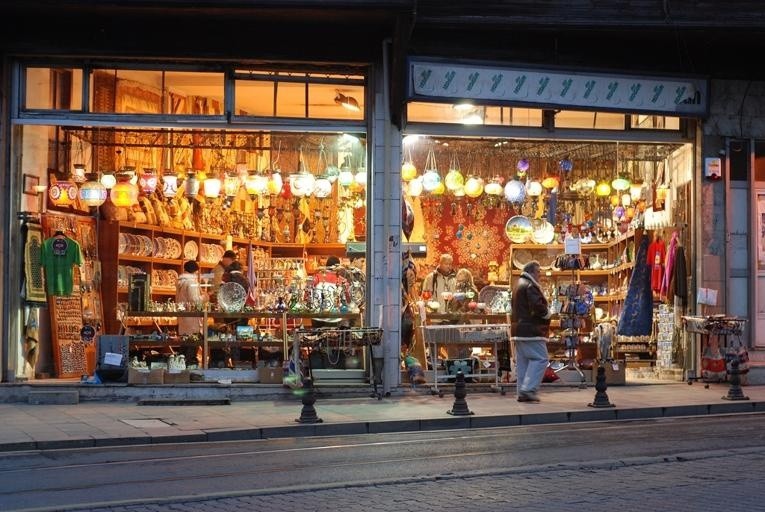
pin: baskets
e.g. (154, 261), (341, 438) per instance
(259, 368), (283, 384)
(128, 368), (190, 384)
(681, 316), (748, 334)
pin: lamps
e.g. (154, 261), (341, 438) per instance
(401, 136), (630, 204)
(334, 89), (360, 112)
(45, 127), (368, 212)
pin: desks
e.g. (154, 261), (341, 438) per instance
(680, 315), (751, 390)
(295, 327), (385, 401)
(412, 311), (512, 398)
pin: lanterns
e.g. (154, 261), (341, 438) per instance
(49, 166), (367, 210)
(401, 162), (644, 208)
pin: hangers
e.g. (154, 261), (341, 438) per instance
(52, 230), (66, 239)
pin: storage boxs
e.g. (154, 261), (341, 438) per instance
(257, 365), (284, 384)
(127, 364), (190, 385)
(590, 358), (626, 384)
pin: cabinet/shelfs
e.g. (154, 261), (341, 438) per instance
(506, 225), (683, 367)
(97, 219), (345, 350)
(124, 307), (365, 382)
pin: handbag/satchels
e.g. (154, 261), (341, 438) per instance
(560, 284), (594, 347)
(555, 254), (590, 270)
(702, 346), (749, 378)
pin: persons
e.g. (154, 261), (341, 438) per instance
(313, 253), (365, 332)
(176, 260), (210, 367)
(510, 260), (553, 402)
(210, 250), (251, 368)
(423, 254), (479, 325)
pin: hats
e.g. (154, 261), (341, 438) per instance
(223, 250), (237, 259)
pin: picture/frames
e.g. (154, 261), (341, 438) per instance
(22, 173), (39, 196)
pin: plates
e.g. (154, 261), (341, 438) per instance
(504, 215), (555, 269)
(478, 286), (502, 311)
(116, 233), (226, 290)
(217, 282), (246, 313)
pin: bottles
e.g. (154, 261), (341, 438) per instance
(592, 253), (601, 269)
(282, 224), (290, 243)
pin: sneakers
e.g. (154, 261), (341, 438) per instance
(517, 390), (540, 402)
